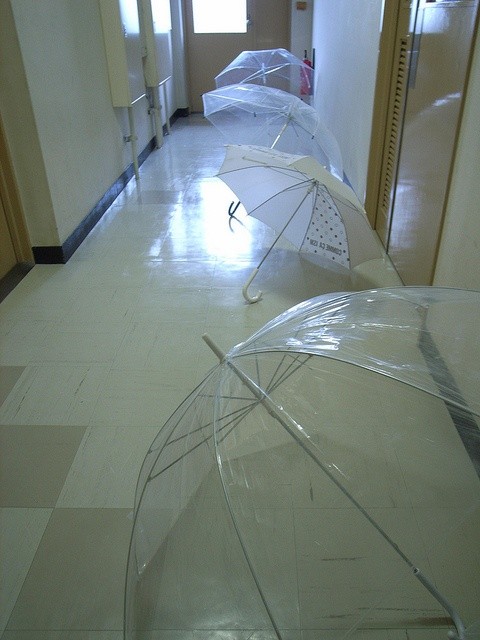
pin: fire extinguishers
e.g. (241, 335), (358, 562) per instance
(299, 49), (313, 96)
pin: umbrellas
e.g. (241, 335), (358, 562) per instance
(202, 83), (343, 214)
(125, 288), (478, 636)
(212, 143), (384, 304)
(213, 49), (316, 92)
